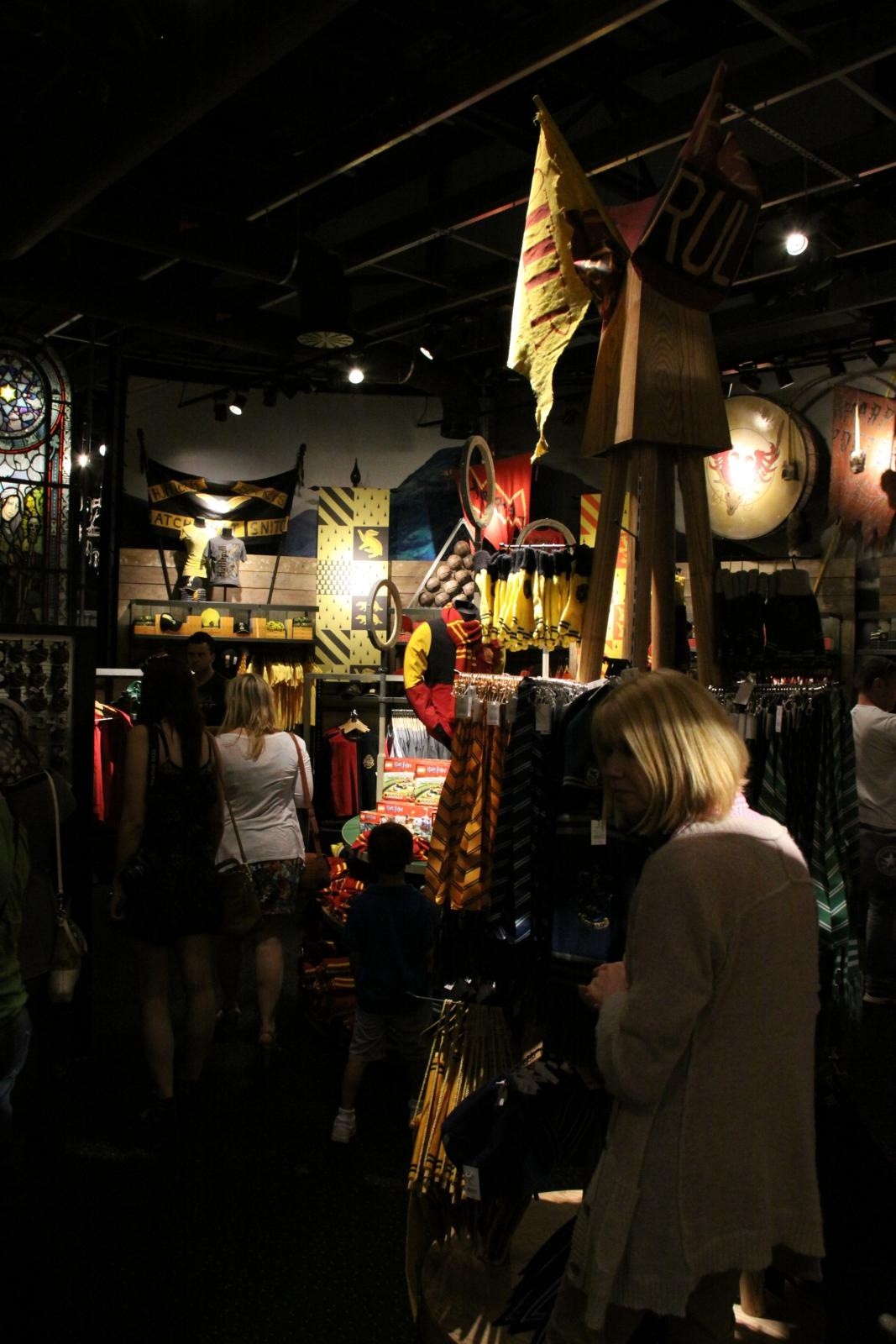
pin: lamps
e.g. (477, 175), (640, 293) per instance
(263, 385), (278, 406)
(229, 395), (249, 414)
(214, 403), (226, 422)
(866, 342), (888, 367)
(722, 382), (732, 398)
(819, 217), (854, 250)
(775, 365), (794, 390)
(825, 350), (846, 377)
(745, 373), (761, 392)
(419, 329), (448, 360)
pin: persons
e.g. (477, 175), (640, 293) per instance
(404, 600), (500, 735)
(548, 667), (826, 1343)
(188, 632), (230, 736)
(0, 790), (31, 1206)
(203, 527), (247, 602)
(849, 655), (896, 1003)
(214, 674), (313, 1049)
(109, 655), (224, 1120)
(180, 517), (216, 588)
(331, 822), (437, 1143)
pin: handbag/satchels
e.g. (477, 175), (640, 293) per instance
(222, 856), (304, 936)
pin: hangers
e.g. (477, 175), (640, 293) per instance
(389, 709), (424, 729)
(338, 718), (370, 734)
(407, 1001), (512, 1202)
(94, 700), (122, 722)
(711, 683), (836, 715)
(454, 673), (589, 706)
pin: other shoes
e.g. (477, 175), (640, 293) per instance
(331, 1115), (356, 1143)
(212, 999), (242, 1025)
(257, 1031), (282, 1058)
(861, 992), (896, 1005)
